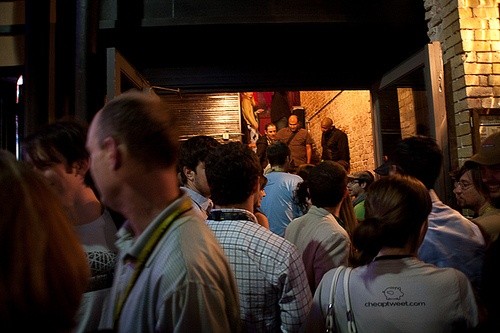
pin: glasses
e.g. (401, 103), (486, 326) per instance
(348, 179), (359, 184)
(454, 180), (478, 190)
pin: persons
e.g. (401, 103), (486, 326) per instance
(0, 91), (500, 333)
(205, 141), (313, 333)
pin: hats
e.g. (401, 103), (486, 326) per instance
(347, 170), (370, 180)
(373, 136), (442, 175)
(464, 130), (500, 168)
